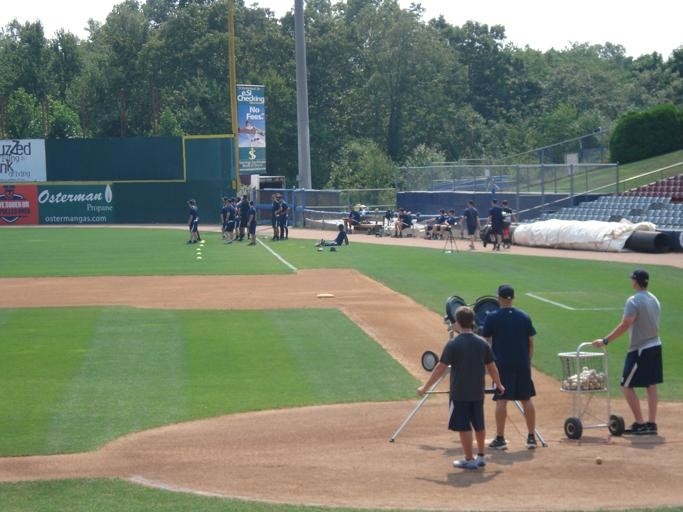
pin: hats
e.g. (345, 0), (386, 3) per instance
(498, 284), (514, 299)
(630, 269), (650, 283)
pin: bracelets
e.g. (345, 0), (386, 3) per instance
(603, 337), (608, 345)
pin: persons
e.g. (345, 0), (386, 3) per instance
(418, 306), (505, 469)
(592, 269), (663, 434)
(186, 200), (200, 243)
(220, 194), (258, 245)
(474, 285), (537, 450)
(480, 200), (516, 250)
(350, 204), (374, 229)
(387, 208), (411, 237)
(462, 200), (479, 248)
(320, 224), (349, 246)
(431, 209), (456, 240)
(272, 193), (289, 240)
(0, 186), (23, 201)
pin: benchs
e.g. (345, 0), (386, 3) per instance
(350, 215), (385, 235)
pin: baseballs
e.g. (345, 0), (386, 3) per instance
(563, 367), (606, 390)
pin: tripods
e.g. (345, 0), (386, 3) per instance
(443, 231), (458, 252)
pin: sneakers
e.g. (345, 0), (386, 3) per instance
(525, 438), (536, 448)
(625, 423), (658, 435)
(486, 439), (507, 450)
(453, 453), (485, 470)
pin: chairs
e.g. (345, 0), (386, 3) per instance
(521, 173), (682, 230)
